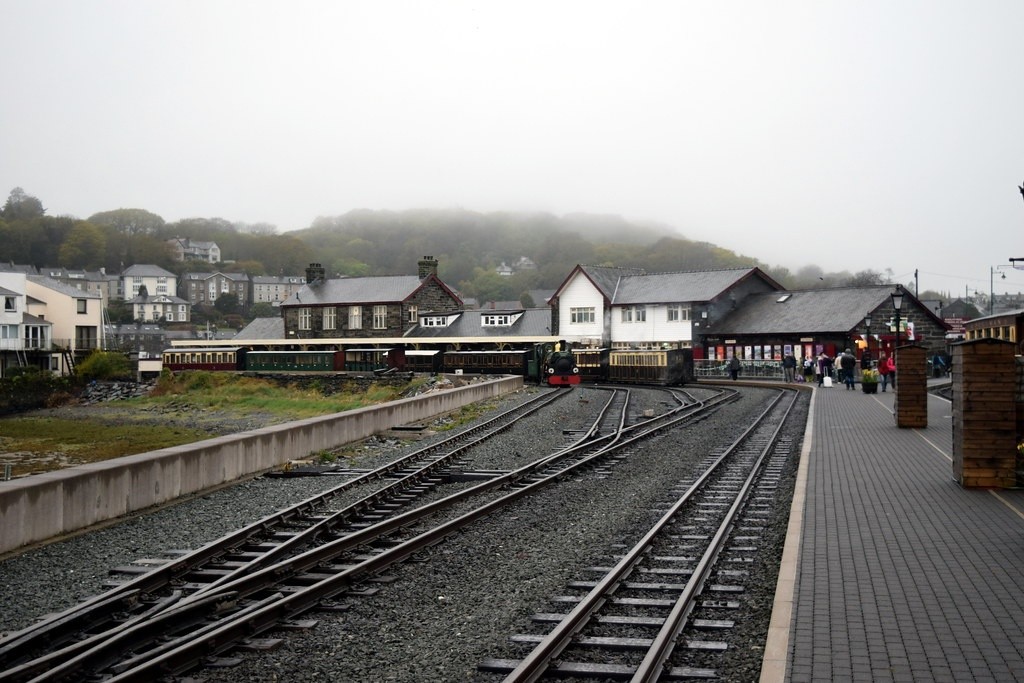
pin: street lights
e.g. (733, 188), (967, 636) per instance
(891, 286), (903, 347)
(967, 289), (979, 321)
(863, 313), (872, 357)
(989, 271), (1006, 315)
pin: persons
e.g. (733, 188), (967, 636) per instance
(860, 347), (872, 371)
(835, 353), (845, 384)
(730, 355), (740, 380)
(887, 352), (895, 392)
(877, 351), (889, 392)
(932, 352), (945, 378)
(841, 348), (856, 390)
(781, 351), (832, 387)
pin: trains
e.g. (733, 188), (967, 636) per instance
(570, 348), (694, 386)
(162, 347), (582, 389)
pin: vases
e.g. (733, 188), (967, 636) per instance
(861, 382), (878, 394)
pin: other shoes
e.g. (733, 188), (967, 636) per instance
(852, 387), (856, 390)
(882, 390), (886, 392)
(846, 387), (849, 390)
(892, 389), (896, 393)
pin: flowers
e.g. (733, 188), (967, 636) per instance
(862, 369), (879, 383)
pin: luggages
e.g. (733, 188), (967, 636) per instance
(818, 383), (821, 387)
(823, 366), (834, 388)
